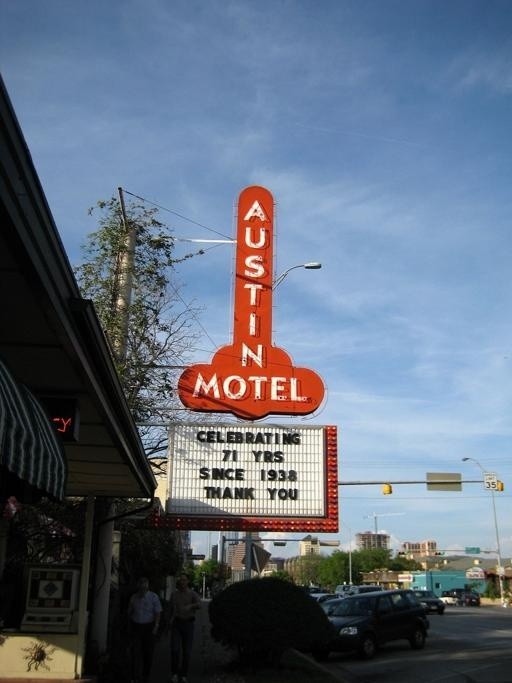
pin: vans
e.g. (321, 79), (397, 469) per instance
(312, 588), (430, 659)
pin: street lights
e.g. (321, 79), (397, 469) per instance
(272, 262), (321, 291)
(460, 455), (505, 605)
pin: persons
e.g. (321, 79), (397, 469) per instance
(118, 576), (160, 683)
(168, 573), (201, 683)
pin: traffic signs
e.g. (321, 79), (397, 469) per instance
(465, 547), (480, 554)
(482, 472), (497, 489)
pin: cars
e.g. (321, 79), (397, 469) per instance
(308, 583), (480, 615)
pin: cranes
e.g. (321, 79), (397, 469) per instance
(363, 510), (406, 533)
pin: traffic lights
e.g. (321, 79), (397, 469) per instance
(435, 552), (444, 555)
(399, 552), (406, 555)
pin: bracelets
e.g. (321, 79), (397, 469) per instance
(155, 624), (160, 627)
